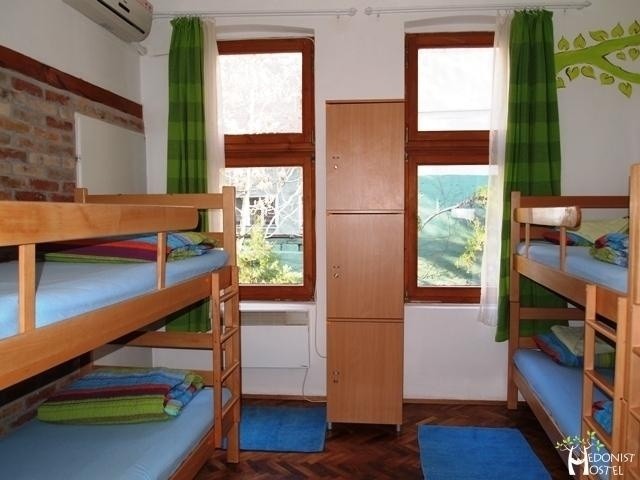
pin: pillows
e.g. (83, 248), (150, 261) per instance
(542, 214), (629, 245)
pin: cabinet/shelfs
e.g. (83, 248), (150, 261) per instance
(325, 98), (404, 433)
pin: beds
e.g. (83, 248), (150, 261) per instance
(0, 185), (240, 480)
(506, 162), (640, 480)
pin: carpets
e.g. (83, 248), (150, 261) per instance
(239, 405), (554, 480)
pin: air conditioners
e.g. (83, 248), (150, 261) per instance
(62, 0), (154, 44)
